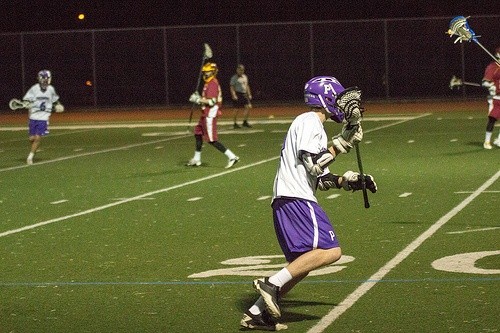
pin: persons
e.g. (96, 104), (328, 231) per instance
(22, 69), (64, 165)
(185, 62), (240, 168)
(228, 65), (253, 129)
(239, 76), (378, 330)
(482, 45), (500, 149)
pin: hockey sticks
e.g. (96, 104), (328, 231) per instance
(9, 98), (56, 111)
(445, 16), (500, 65)
(335, 86), (371, 210)
(187, 42), (213, 135)
(449, 75), (480, 90)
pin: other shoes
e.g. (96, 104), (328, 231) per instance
(234, 122), (252, 129)
(483, 138), (500, 149)
(26, 159), (32, 164)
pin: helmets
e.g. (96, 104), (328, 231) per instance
(38, 71), (52, 85)
(495, 47), (500, 58)
(304, 76), (345, 122)
(202, 63), (217, 82)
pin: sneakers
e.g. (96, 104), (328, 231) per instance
(184, 159), (202, 167)
(224, 156), (240, 169)
(253, 277), (282, 318)
(241, 309), (275, 330)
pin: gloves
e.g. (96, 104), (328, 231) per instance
(331, 122), (364, 154)
(342, 171), (378, 193)
(489, 86), (496, 95)
(189, 91), (202, 105)
(25, 102), (33, 109)
(55, 105), (64, 112)
